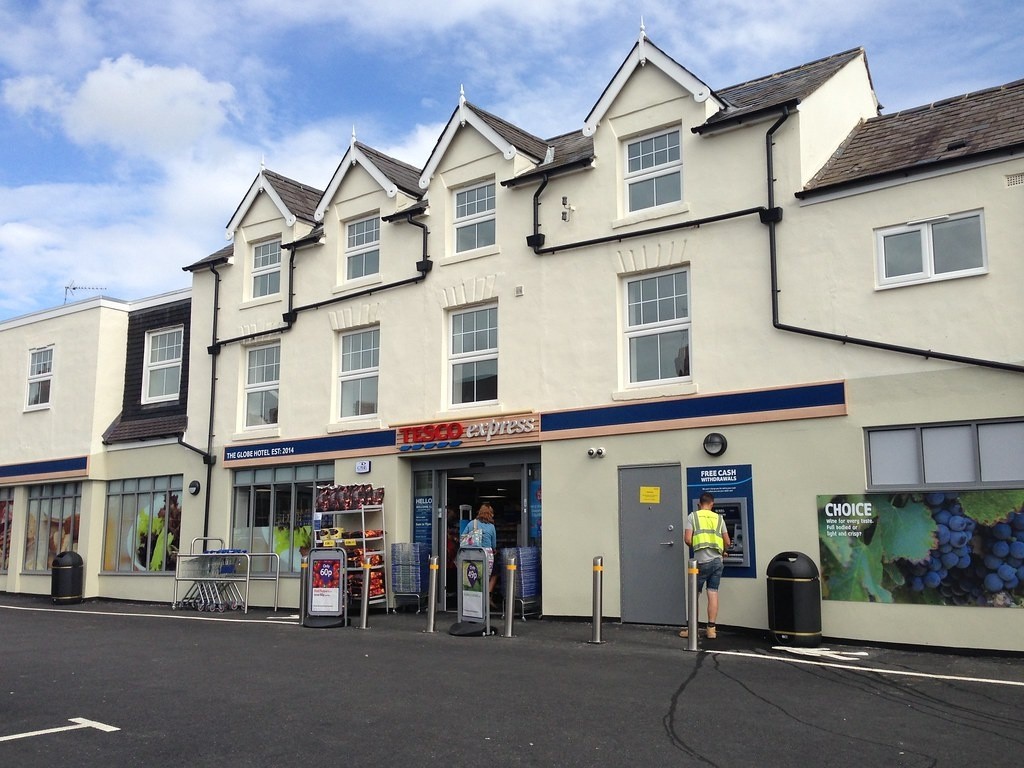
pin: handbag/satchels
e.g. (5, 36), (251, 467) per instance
(459, 519), (483, 547)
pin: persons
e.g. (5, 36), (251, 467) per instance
(459, 505), (499, 608)
(679, 492), (731, 638)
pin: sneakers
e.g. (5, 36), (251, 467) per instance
(679, 629), (700, 638)
(705, 626), (717, 639)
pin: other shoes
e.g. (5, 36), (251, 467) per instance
(489, 593), (499, 610)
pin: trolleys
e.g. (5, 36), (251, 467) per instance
(178, 549), (248, 613)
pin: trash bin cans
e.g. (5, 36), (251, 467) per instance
(50, 550), (84, 605)
(765, 551), (823, 648)
